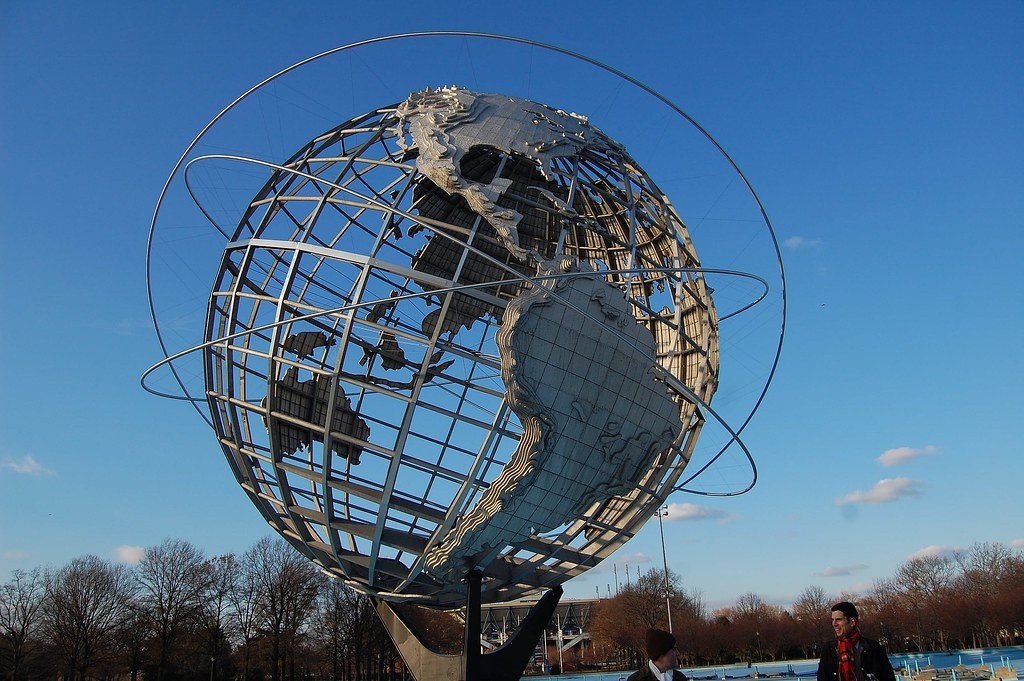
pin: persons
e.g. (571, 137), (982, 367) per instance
(816, 601), (896, 681)
(627, 628), (687, 681)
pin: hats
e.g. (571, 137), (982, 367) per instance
(646, 626), (677, 662)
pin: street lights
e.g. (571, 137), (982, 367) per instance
(377, 651), (381, 681)
(653, 503), (674, 636)
(210, 657), (215, 680)
(140, 646), (148, 680)
